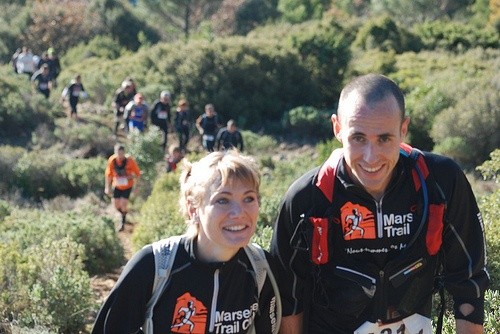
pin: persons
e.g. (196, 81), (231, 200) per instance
(267, 73), (491, 334)
(104, 143), (141, 233)
(111, 79), (244, 173)
(90, 144), (283, 334)
(11, 46), (86, 120)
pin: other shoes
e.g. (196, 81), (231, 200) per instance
(119, 226), (124, 232)
